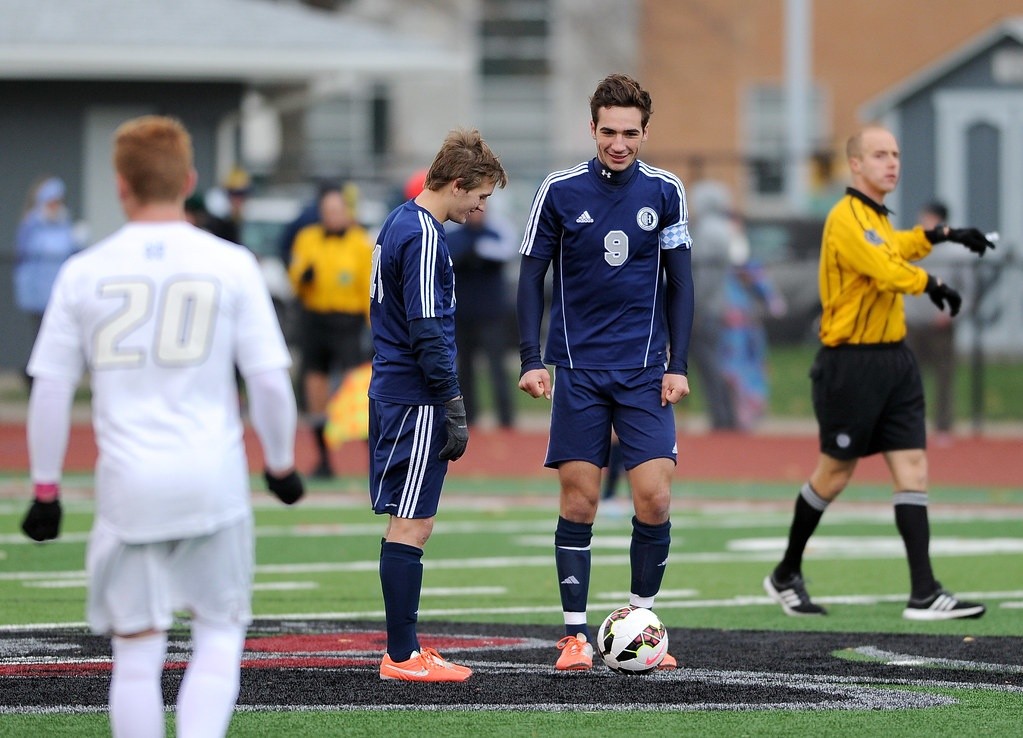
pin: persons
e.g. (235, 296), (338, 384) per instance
(13, 177), (90, 386)
(763, 124), (996, 621)
(368, 122), (507, 682)
(518, 74), (694, 669)
(887, 202), (960, 432)
(22, 115), (306, 738)
(179, 165), (786, 479)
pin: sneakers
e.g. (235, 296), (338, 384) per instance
(554, 638), (592, 668)
(904, 592), (984, 620)
(765, 570), (828, 616)
(380, 647), (473, 682)
(659, 654), (676, 668)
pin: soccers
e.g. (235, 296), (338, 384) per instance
(596, 608), (668, 675)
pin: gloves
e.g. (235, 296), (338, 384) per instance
(21, 499), (64, 540)
(948, 228), (995, 260)
(925, 275), (961, 316)
(265, 470), (305, 505)
(439, 398), (470, 460)
